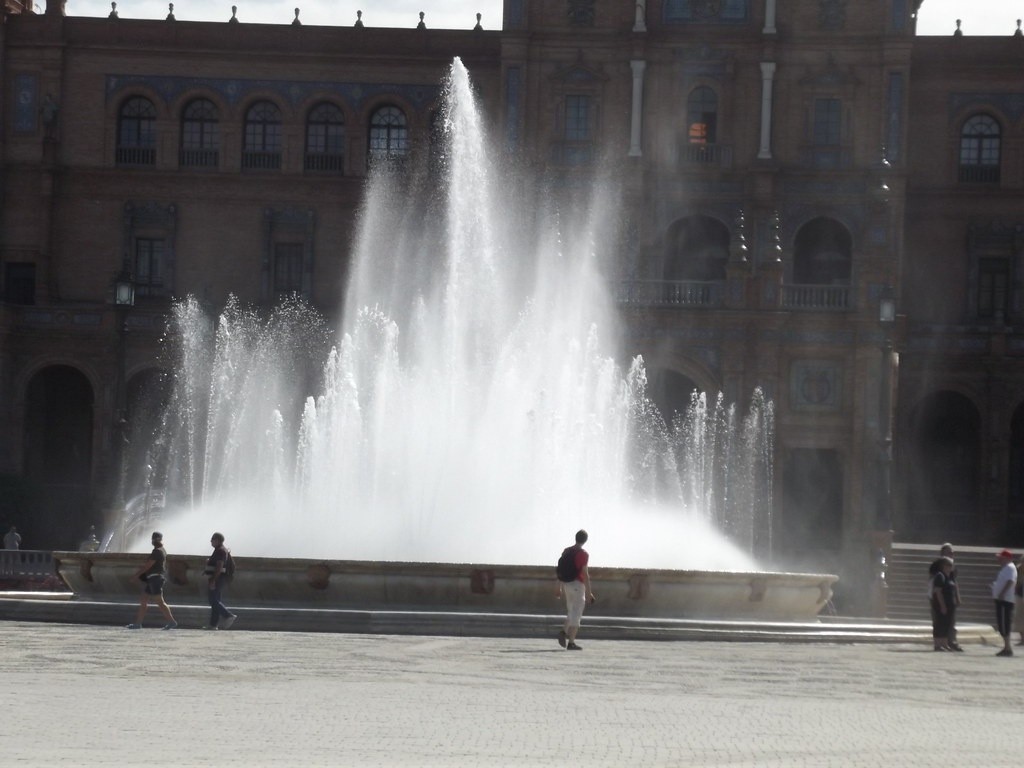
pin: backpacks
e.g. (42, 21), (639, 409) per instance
(556, 546), (585, 582)
(226, 550), (236, 584)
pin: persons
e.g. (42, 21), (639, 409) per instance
(1014, 555), (1024, 646)
(3, 526), (21, 573)
(126, 532), (177, 630)
(202, 533), (237, 631)
(990, 551), (1018, 657)
(554, 530), (596, 650)
(928, 542), (963, 652)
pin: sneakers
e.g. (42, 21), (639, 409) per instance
(557, 630), (567, 648)
(567, 642), (583, 650)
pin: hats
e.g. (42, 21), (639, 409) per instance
(994, 549), (1013, 560)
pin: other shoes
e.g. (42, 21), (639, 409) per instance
(995, 649), (1014, 656)
(934, 643), (963, 651)
(202, 625), (218, 629)
(126, 624), (142, 628)
(162, 621), (177, 630)
(224, 614), (237, 629)
